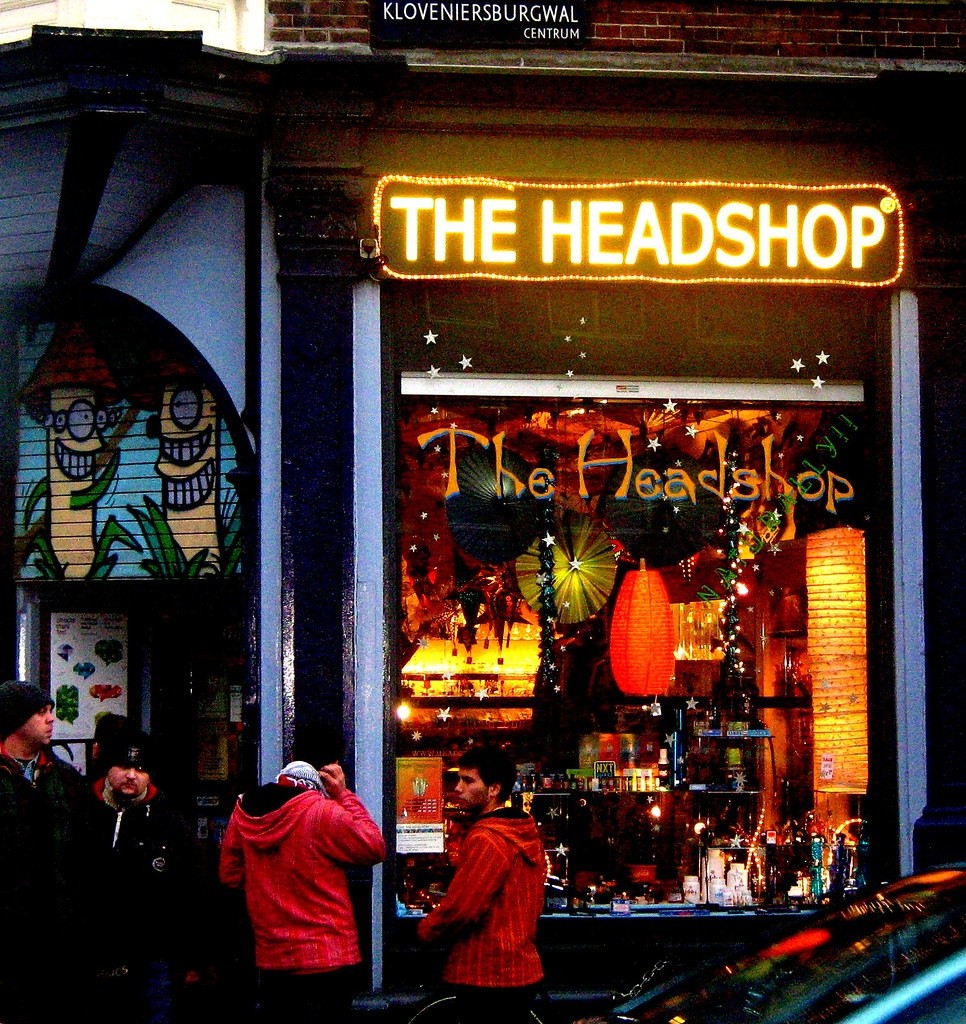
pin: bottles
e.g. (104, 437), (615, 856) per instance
(682, 850), (752, 907)
(514, 749), (672, 792)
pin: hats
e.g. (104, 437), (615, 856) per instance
(0, 681), (54, 741)
(99, 726), (154, 771)
(281, 761), (325, 791)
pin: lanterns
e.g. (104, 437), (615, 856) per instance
(608, 569), (675, 695)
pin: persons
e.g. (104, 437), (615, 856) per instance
(217, 720), (388, 1024)
(0, 680), (199, 1024)
(418, 747), (546, 1024)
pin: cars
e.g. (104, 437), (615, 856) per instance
(571, 860), (965, 1023)
